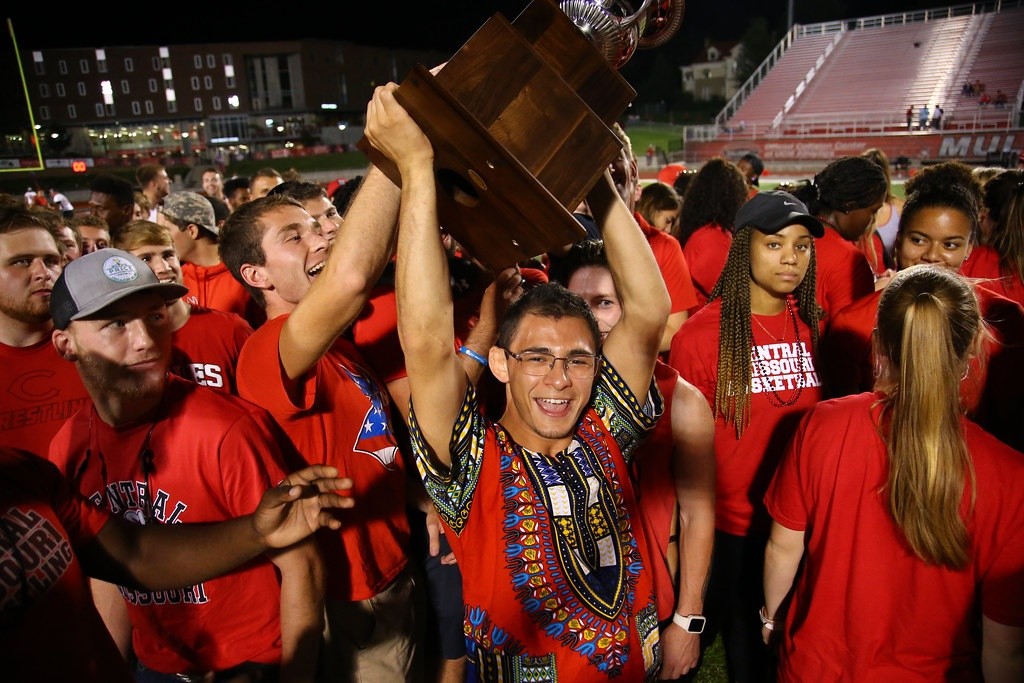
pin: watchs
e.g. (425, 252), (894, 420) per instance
(672, 609), (707, 634)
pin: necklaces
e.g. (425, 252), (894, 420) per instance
(743, 288), (807, 410)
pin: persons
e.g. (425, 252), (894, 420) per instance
(0, 144), (1024, 683)
(963, 78), (1007, 107)
(919, 103), (929, 128)
(932, 104), (944, 130)
(905, 104), (913, 130)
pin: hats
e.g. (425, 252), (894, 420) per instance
(158, 191), (221, 236)
(734, 189), (825, 239)
(50, 248), (189, 330)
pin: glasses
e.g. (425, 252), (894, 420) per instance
(503, 345), (602, 380)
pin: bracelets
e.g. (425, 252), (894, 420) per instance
(457, 345), (490, 368)
(758, 605), (786, 628)
